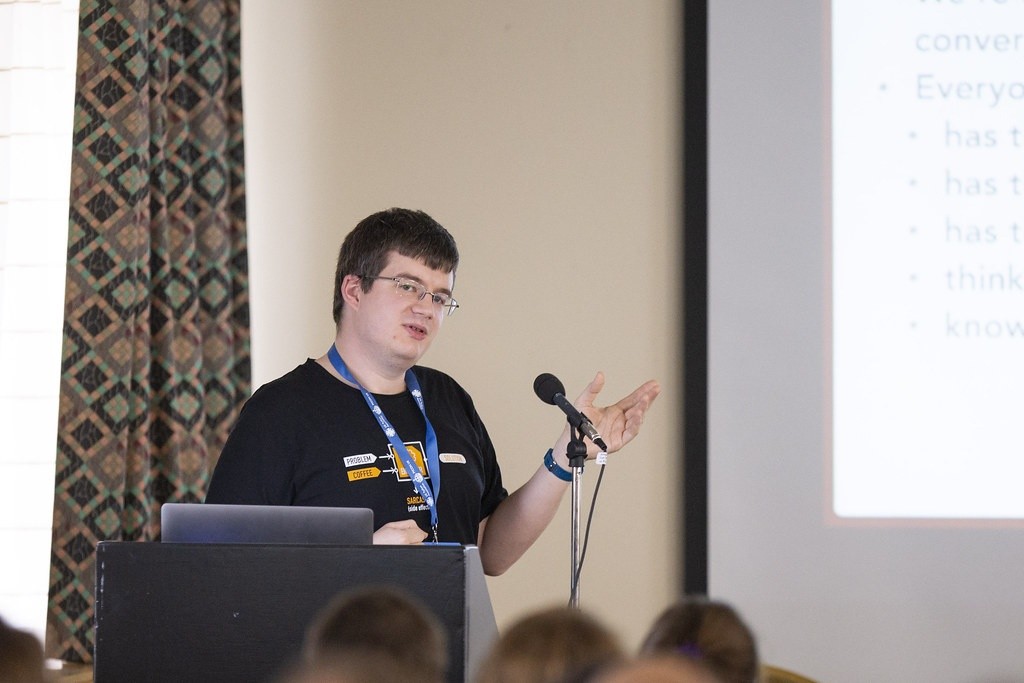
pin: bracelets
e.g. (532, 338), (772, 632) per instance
(544, 448), (573, 483)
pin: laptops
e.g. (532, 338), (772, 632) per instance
(161, 503), (374, 547)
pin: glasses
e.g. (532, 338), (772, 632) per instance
(363, 275), (459, 316)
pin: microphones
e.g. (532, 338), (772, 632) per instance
(534, 373), (607, 450)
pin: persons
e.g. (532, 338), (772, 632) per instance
(288, 582), (765, 683)
(203, 208), (661, 576)
